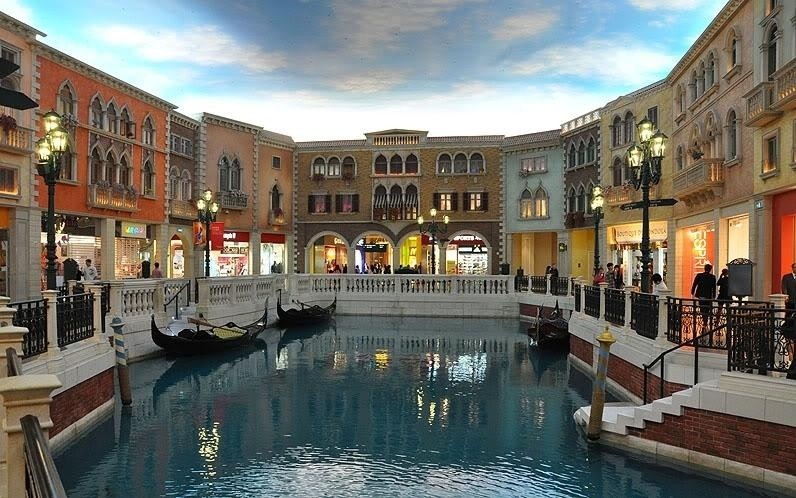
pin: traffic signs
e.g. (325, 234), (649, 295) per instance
(621, 201), (643, 211)
(649, 198), (679, 207)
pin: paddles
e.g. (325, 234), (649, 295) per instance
(188, 317), (244, 335)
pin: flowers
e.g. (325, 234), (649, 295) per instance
(0, 112), (16, 136)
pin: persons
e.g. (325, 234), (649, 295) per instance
(326, 259), (422, 274)
(652, 274), (670, 305)
(270, 261), (280, 273)
(551, 264), (558, 295)
(136, 262), (142, 279)
(152, 263), (163, 278)
(592, 268), (605, 284)
(544, 266), (552, 294)
(604, 262), (614, 288)
(714, 269), (732, 326)
(613, 265), (623, 289)
(277, 262), (283, 273)
(782, 316), (795, 379)
(781, 263), (796, 307)
(142, 260), (150, 278)
(81, 258), (101, 280)
(690, 263), (716, 327)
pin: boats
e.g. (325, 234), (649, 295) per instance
(152, 337), (267, 414)
(529, 299), (569, 343)
(277, 323), (337, 362)
(150, 297), (270, 350)
(276, 296), (339, 324)
(528, 346), (567, 386)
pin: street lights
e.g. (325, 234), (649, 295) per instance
(626, 116), (669, 294)
(199, 353), (224, 483)
(416, 208), (449, 274)
(589, 185), (605, 273)
(32, 108), (68, 290)
(195, 187), (219, 276)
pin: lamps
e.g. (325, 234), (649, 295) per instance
(559, 242), (567, 252)
